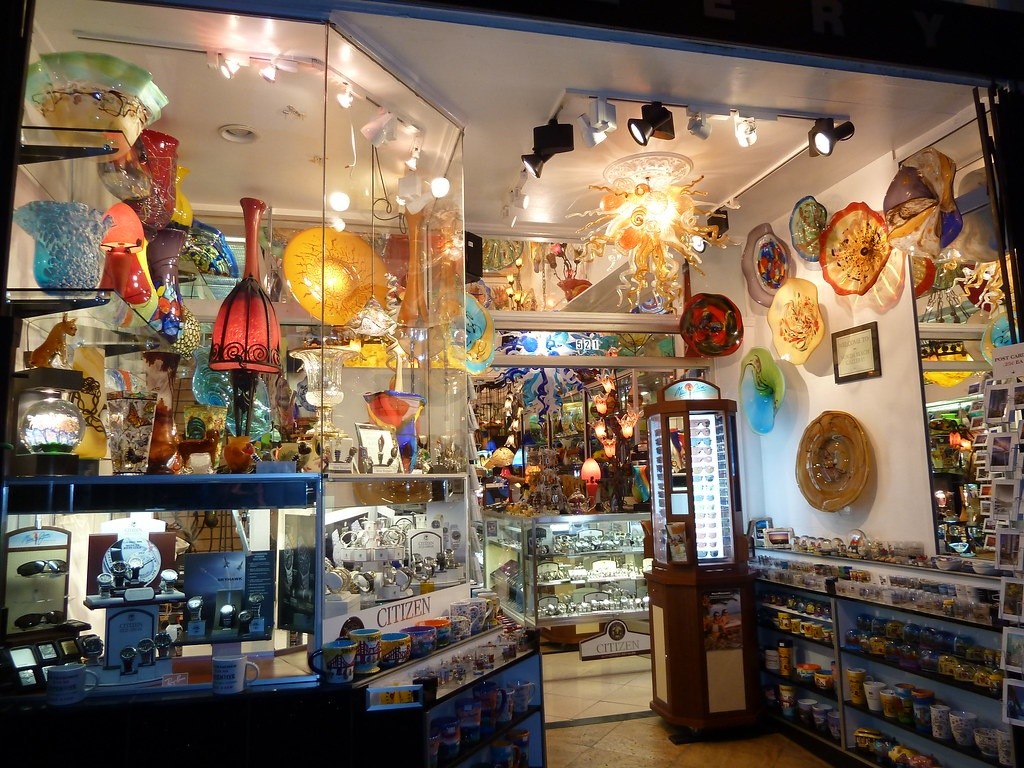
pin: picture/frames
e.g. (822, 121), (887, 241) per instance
(983, 381), (1024, 727)
(831, 322), (881, 383)
(970, 416), (995, 550)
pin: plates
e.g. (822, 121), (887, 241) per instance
(680, 149), (963, 511)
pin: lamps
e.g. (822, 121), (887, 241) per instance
(505, 243), (547, 311)
(215, 52), (437, 214)
(210, 271), (280, 470)
(568, 153), (742, 318)
(590, 367), (638, 512)
(919, 289), (973, 386)
(507, 96), (758, 210)
(808, 117), (856, 158)
(581, 459), (603, 514)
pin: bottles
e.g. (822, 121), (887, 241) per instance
(13, 126), (286, 372)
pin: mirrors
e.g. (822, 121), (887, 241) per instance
(908, 182), (1024, 560)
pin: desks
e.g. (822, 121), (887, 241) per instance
(643, 571), (757, 728)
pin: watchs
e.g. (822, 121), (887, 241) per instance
(220, 592), (265, 623)
(119, 633), (172, 671)
(161, 569), (178, 593)
(545, 597), (649, 614)
(324, 548), (454, 593)
(78, 633), (104, 658)
(283, 534), (311, 590)
(96, 557), (142, 599)
(187, 596), (203, 620)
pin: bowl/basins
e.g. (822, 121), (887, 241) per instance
(972, 561), (998, 575)
(934, 558), (961, 570)
(25, 50), (167, 161)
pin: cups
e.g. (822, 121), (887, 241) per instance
(416, 616), (471, 647)
(462, 599), (493, 632)
(107, 392), (157, 476)
(48, 666), (98, 705)
(212, 656), (260, 695)
(380, 633), (411, 666)
(450, 602), (479, 636)
(308, 640), (357, 683)
(184, 406), (228, 473)
(749, 520), (1024, 768)
(415, 682), (535, 768)
(470, 579), (500, 625)
(399, 626), (437, 659)
(350, 629), (381, 674)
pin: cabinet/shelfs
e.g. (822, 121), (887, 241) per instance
(747, 554), (1024, 768)
(480, 508), (652, 632)
(0, 0), (547, 768)
